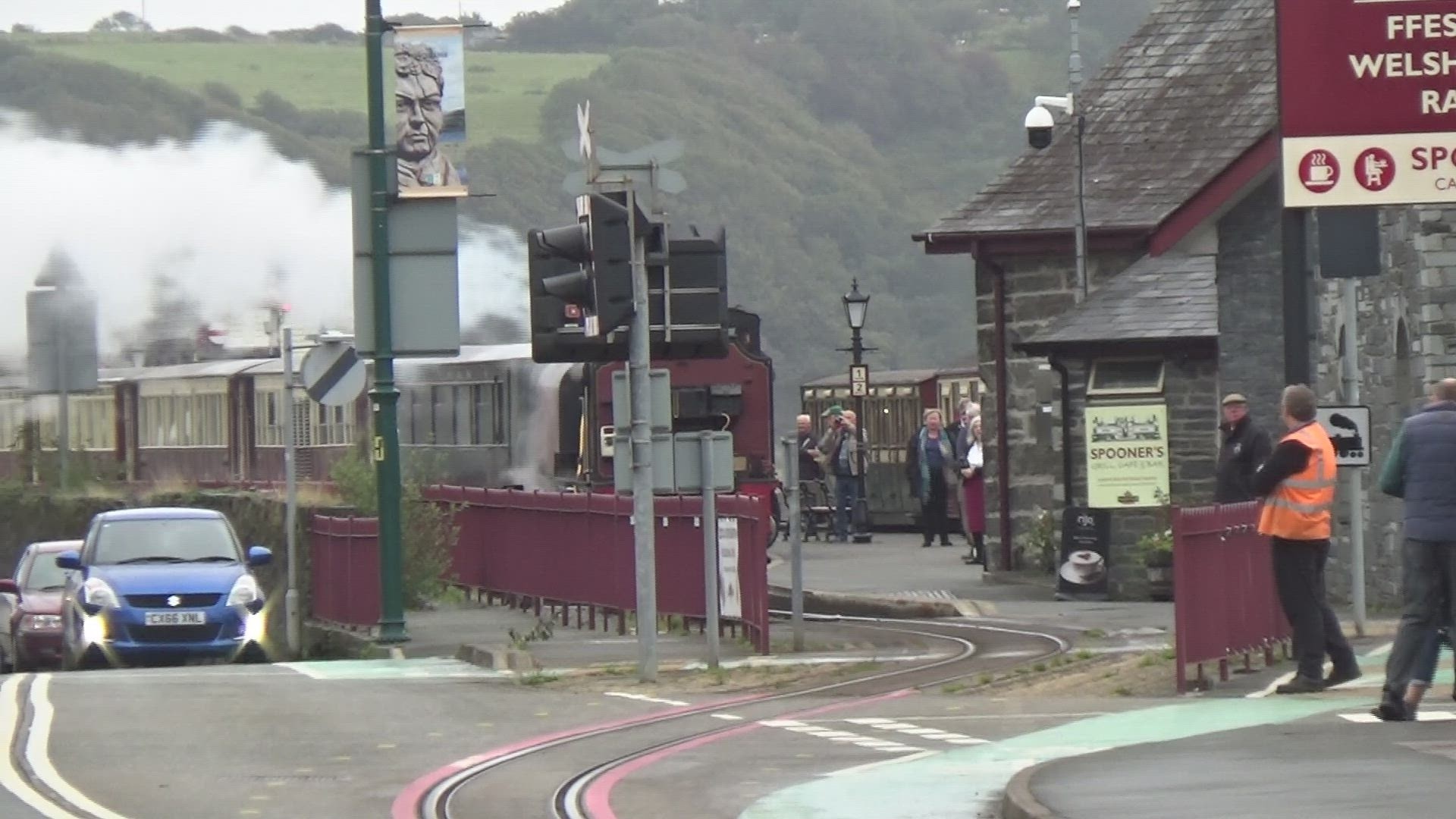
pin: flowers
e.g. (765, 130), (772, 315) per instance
(1132, 488), (1174, 561)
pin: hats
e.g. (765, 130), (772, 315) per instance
(1221, 394), (1246, 404)
(820, 405), (844, 417)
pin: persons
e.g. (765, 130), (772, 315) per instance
(780, 404), (870, 544)
(1372, 378), (1456, 723)
(905, 397), (985, 565)
(1213, 394), (1271, 503)
(393, 40), (462, 188)
(1251, 384), (1362, 694)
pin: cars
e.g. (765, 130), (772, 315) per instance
(55, 506), (274, 667)
(1, 539), (85, 671)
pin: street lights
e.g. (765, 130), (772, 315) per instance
(836, 277), (879, 544)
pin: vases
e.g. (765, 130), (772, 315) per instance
(1142, 548), (1175, 600)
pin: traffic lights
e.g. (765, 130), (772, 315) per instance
(533, 193), (634, 336)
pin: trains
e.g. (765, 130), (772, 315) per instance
(1, 307), (783, 562)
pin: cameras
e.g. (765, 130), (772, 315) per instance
(833, 417), (843, 425)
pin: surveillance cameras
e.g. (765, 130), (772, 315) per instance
(1022, 91), (1074, 151)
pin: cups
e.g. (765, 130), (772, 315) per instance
(1070, 550), (1102, 577)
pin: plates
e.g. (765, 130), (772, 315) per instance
(1061, 562), (1106, 584)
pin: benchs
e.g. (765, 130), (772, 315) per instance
(777, 477), (854, 543)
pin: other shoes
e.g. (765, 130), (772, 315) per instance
(965, 557), (984, 565)
(941, 540), (951, 546)
(961, 554), (972, 559)
(1381, 691), (1404, 720)
(922, 540), (931, 547)
(1323, 661), (1363, 687)
(1371, 699), (1416, 722)
(1276, 672), (1323, 693)
